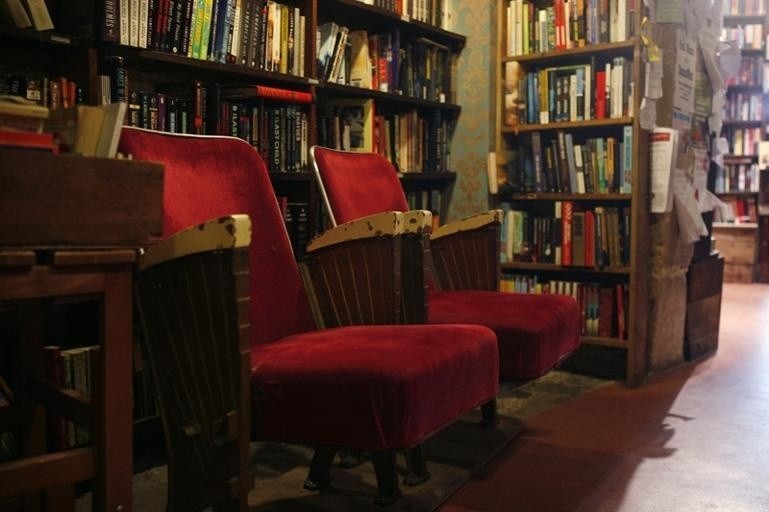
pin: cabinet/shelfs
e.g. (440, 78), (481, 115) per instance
(708, 0), (769, 286)
(493, 1), (652, 390)
(0, 0), (469, 484)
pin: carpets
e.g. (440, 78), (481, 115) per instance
(59, 360), (624, 512)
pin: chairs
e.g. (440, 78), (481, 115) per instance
(306, 138), (588, 487)
(113, 124), (506, 512)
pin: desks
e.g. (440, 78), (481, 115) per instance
(0, 148), (178, 511)
